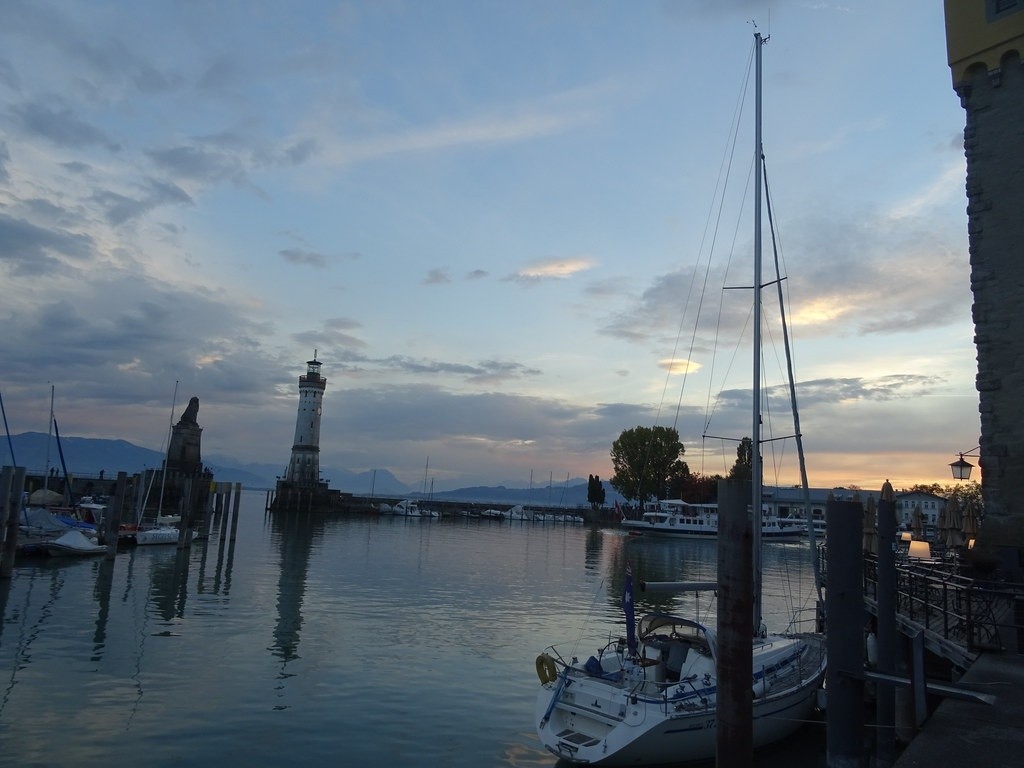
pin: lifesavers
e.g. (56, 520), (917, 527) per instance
(535, 654), (559, 684)
(672, 512), (676, 516)
(706, 514), (710, 518)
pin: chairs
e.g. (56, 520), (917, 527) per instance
(634, 646), (663, 694)
(584, 655), (623, 682)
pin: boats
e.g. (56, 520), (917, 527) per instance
(620, 499), (803, 541)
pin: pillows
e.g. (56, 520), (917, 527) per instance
(636, 658), (661, 667)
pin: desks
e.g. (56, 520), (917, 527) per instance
(899, 556), (994, 645)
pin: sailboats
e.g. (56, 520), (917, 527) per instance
(0, 381), (198, 554)
(536, 31), (826, 766)
